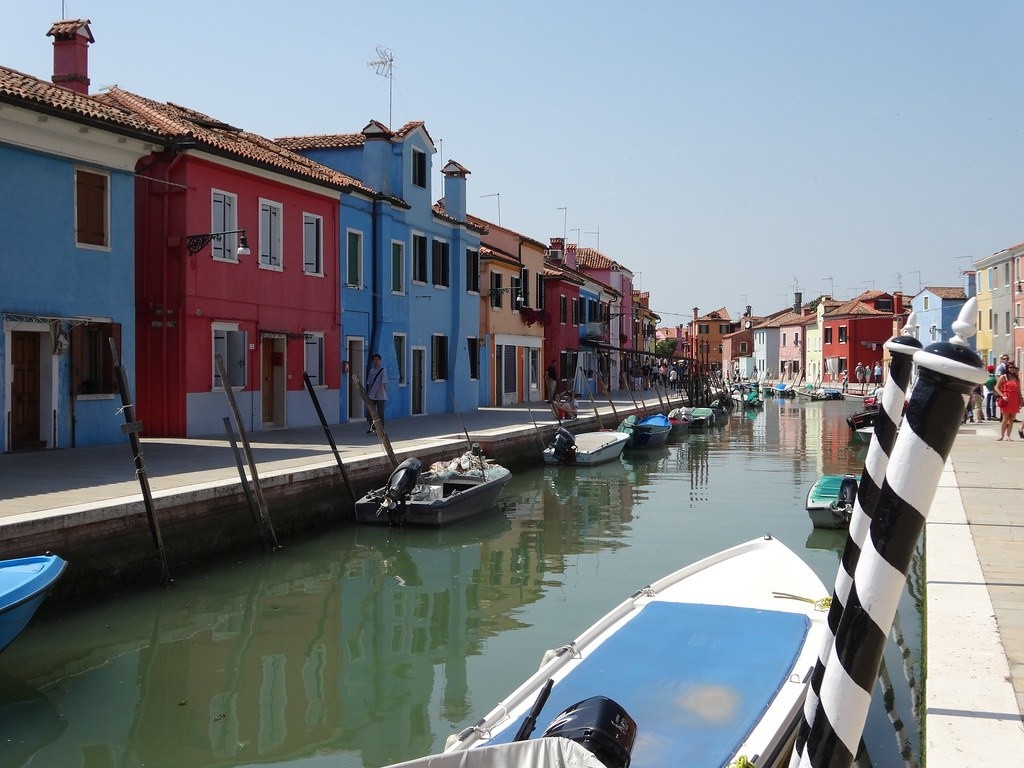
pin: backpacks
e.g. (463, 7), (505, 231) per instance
(858, 366), (863, 374)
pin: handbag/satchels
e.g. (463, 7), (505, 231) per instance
(669, 375), (674, 382)
(996, 395), (1007, 407)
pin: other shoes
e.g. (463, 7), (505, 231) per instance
(964, 419), (966, 425)
(1013, 419), (1021, 422)
(1019, 431), (1024, 438)
(987, 417), (991, 420)
(969, 419), (975, 422)
(367, 427), (376, 433)
(571, 416), (577, 421)
(547, 401), (553, 404)
(996, 437), (1003, 441)
(993, 417), (999, 421)
(977, 420), (983, 423)
(1006, 438), (1013, 442)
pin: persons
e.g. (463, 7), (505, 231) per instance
(552, 390), (578, 420)
(960, 354), (1024, 442)
(545, 360), (557, 404)
(855, 362), (871, 385)
(631, 357), (689, 390)
(366, 355), (387, 433)
(874, 361), (882, 386)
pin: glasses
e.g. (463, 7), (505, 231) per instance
(1009, 366), (1015, 368)
(557, 396), (560, 398)
(1001, 358), (1009, 360)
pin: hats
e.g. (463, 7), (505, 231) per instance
(858, 362), (862, 365)
(987, 365), (995, 371)
(866, 366), (870, 370)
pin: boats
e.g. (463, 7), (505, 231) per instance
(617, 412), (672, 446)
(382, 532), (832, 768)
(0, 551), (68, 651)
(542, 427), (631, 466)
(843, 393), (909, 443)
(805, 473), (862, 528)
(731, 367), (842, 408)
(668, 408), (693, 432)
(355, 443), (512, 527)
